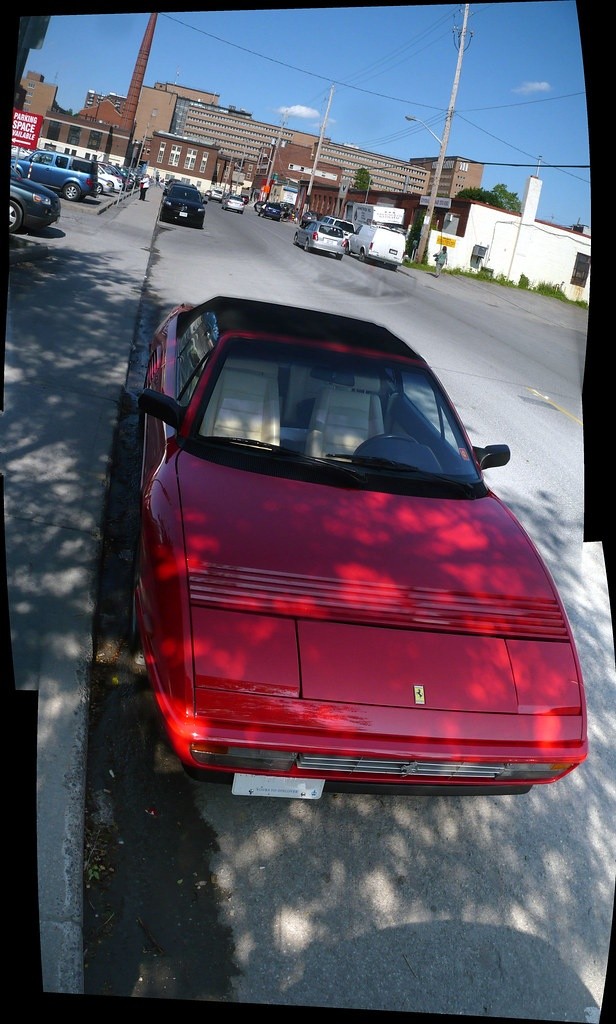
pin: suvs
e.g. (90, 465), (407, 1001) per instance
(209, 187), (224, 203)
(11, 148), (99, 202)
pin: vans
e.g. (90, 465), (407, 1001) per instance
(319, 214), (356, 242)
(345, 223), (406, 271)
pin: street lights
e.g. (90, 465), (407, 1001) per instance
(404, 114), (446, 264)
(260, 153), (275, 203)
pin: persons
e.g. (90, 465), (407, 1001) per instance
(138, 174), (150, 201)
(433, 246), (447, 278)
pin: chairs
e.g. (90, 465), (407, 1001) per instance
(286, 363), (386, 463)
(198, 353), (280, 446)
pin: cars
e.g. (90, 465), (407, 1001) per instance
(137, 296), (591, 800)
(293, 220), (348, 260)
(158, 178), (208, 230)
(222, 192), (249, 214)
(254, 201), (295, 222)
(9, 166), (62, 234)
(302, 211), (323, 224)
(205, 188), (212, 196)
(92, 160), (143, 195)
